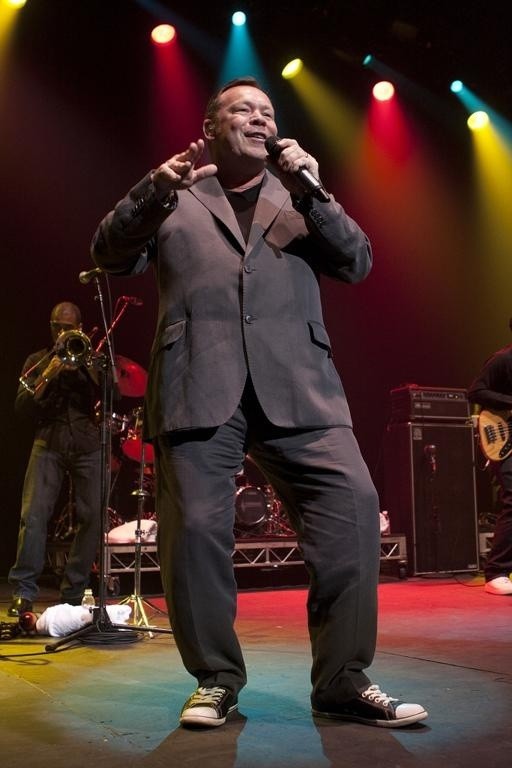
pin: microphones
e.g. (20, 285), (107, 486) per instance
(122, 296), (144, 306)
(265, 136), (330, 203)
(79, 267), (102, 284)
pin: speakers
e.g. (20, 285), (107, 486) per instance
(382, 422), (480, 576)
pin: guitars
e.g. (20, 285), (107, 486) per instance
(476, 405), (512, 465)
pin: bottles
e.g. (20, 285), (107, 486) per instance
(382, 510), (391, 535)
(81, 588), (96, 606)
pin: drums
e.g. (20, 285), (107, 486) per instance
(120, 424), (155, 465)
(47, 502), (158, 582)
(234, 485), (272, 537)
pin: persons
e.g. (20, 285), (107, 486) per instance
(109, 448), (137, 519)
(7, 302), (108, 618)
(469, 317), (512, 595)
(91, 78), (429, 729)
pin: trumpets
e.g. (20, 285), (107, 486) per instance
(18, 326), (100, 397)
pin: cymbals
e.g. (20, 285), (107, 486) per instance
(112, 353), (149, 398)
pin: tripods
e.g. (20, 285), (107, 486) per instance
(45, 286), (174, 652)
(116, 428), (169, 638)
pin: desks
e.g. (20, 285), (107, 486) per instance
(47, 533), (408, 593)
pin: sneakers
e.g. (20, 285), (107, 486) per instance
(483, 576), (512, 596)
(309, 684), (428, 727)
(8, 598), (35, 616)
(178, 685), (239, 726)
